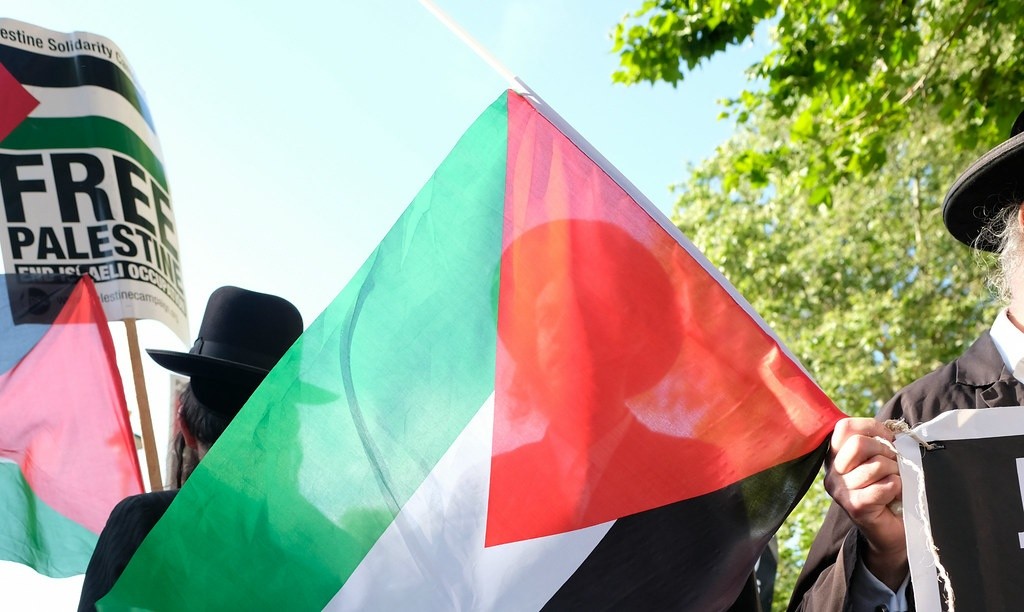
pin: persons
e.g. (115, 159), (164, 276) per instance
(491, 218), (777, 612)
(76, 288), (365, 612)
(784, 102), (1024, 612)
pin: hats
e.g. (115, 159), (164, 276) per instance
(941, 107), (1023, 253)
(487, 216), (683, 398)
(144, 285), (339, 406)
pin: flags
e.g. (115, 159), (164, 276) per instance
(93, 82), (856, 612)
(0, 272), (145, 580)
